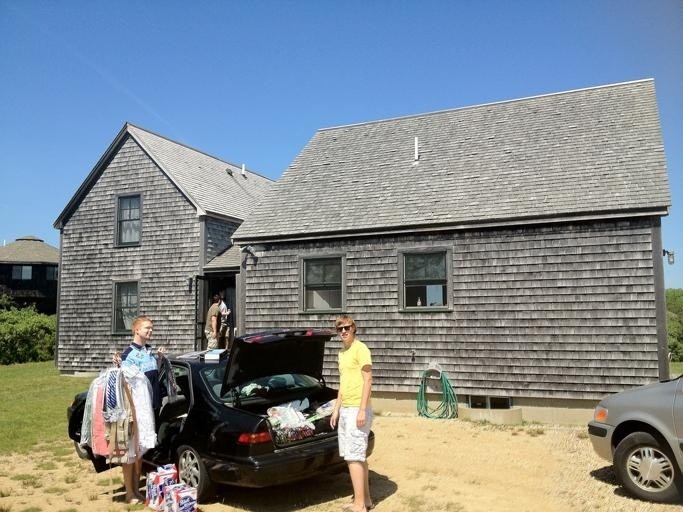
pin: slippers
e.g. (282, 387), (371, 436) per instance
(343, 501), (375, 509)
(126, 497), (143, 505)
(344, 506), (368, 512)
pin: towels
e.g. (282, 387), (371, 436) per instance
(426, 285), (443, 306)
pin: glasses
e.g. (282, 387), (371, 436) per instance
(336, 325), (350, 332)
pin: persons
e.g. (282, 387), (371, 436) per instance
(204, 295), (222, 350)
(219, 291), (231, 348)
(112, 317), (159, 504)
(330, 316), (375, 512)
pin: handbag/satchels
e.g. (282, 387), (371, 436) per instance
(314, 416), (338, 434)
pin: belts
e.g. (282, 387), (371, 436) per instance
(114, 375), (140, 473)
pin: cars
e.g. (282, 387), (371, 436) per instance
(586, 374), (682, 503)
(66, 328), (375, 503)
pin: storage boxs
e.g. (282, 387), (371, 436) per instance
(145, 464), (177, 511)
(165, 484), (197, 512)
